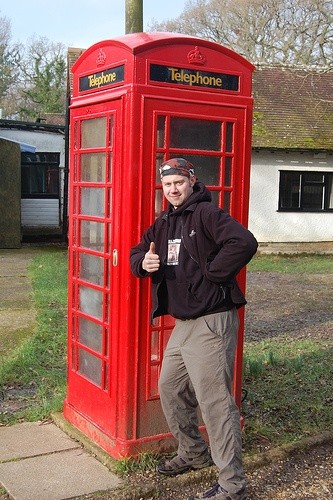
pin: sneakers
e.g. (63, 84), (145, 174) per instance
(158, 453), (211, 475)
(188, 482), (249, 500)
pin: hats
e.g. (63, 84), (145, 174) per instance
(159, 157), (195, 177)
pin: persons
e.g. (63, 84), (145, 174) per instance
(129, 157), (260, 500)
